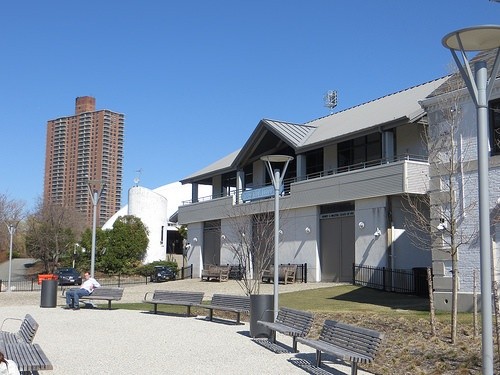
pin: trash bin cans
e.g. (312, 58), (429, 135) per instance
(40, 279), (57, 308)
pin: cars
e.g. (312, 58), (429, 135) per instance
(150, 266), (176, 282)
(53, 267), (82, 286)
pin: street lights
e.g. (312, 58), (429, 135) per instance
(85, 180), (107, 279)
(5, 220), (19, 290)
(260, 155), (294, 322)
(441, 26), (500, 375)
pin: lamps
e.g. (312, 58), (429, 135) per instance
(358, 222), (365, 230)
(304, 226), (311, 234)
(374, 226), (381, 237)
(241, 232), (246, 237)
(278, 229), (284, 235)
(221, 234), (226, 240)
(193, 237), (198, 242)
(436, 221), (447, 231)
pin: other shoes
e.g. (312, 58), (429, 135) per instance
(64, 305), (70, 308)
(73, 306), (80, 310)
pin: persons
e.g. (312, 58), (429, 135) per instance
(0, 352), (20, 375)
(61, 272), (101, 310)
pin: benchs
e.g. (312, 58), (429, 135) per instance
(143, 289), (205, 317)
(62, 287), (124, 310)
(294, 319), (382, 375)
(4, 343), (54, 372)
(198, 294), (250, 324)
(201, 263), (230, 283)
(261, 266), (298, 285)
(0, 313), (39, 360)
(251, 307), (315, 353)
